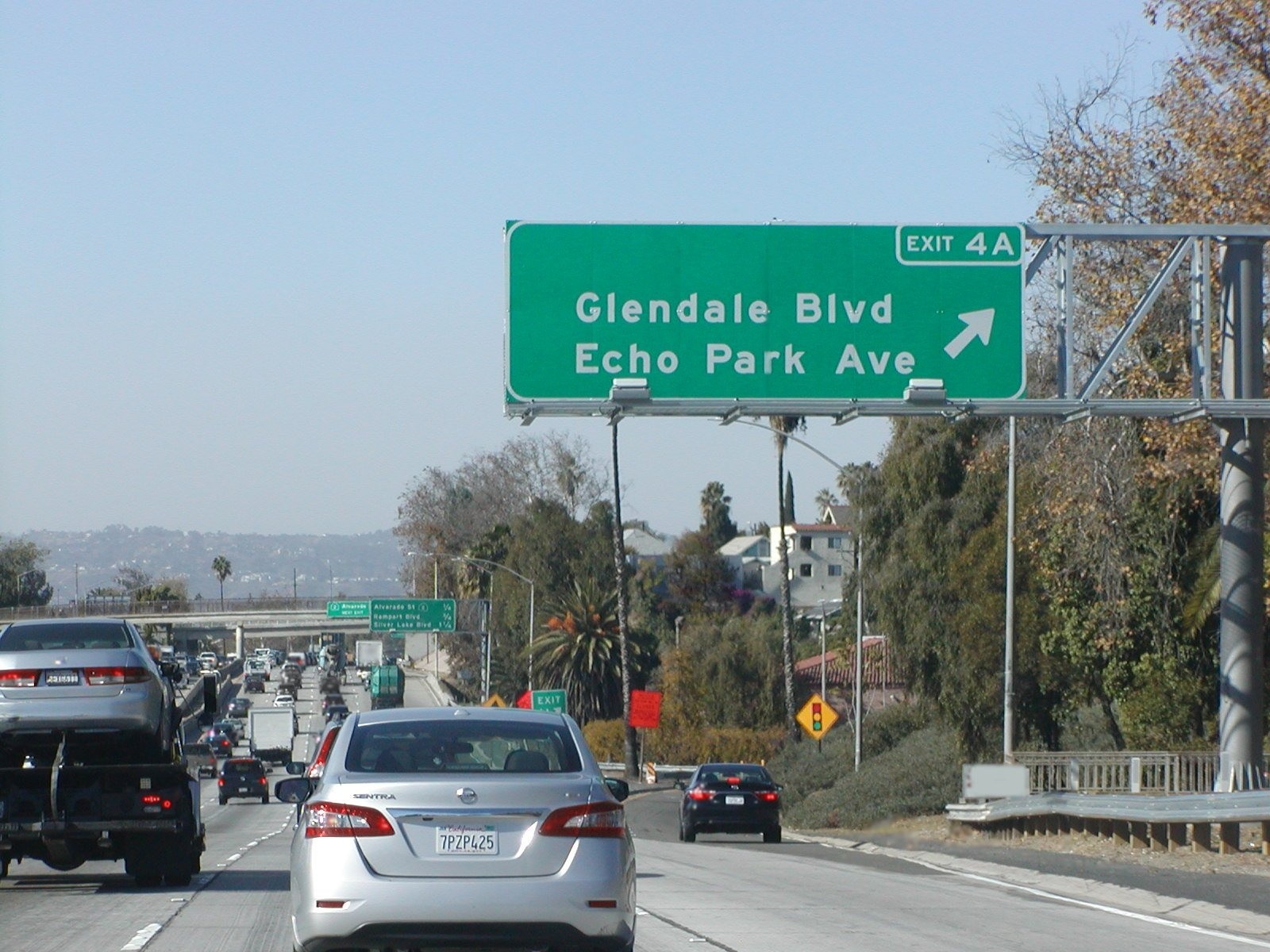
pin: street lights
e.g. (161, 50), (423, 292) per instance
(408, 550), (535, 702)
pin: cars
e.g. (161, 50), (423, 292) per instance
(244, 677), (266, 694)
(217, 758), (274, 806)
(275, 651), (308, 734)
(273, 706), (639, 952)
(679, 762), (785, 844)
(227, 697), (254, 718)
(318, 673), (351, 725)
(161, 651), (236, 691)
(197, 717), (246, 778)
(0, 617), (184, 768)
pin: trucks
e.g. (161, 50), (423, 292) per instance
(0, 723), (207, 887)
(159, 646), (175, 659)
(355, 639), (384, 671)
(245, 706), (300, 765)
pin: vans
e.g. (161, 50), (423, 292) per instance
(242, 647), (278, 682)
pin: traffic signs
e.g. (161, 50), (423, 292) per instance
(324, 598), (459, 634)
(502, 220), (1025, 409)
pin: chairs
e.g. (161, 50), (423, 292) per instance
(503, 750), (550, 771)
(91, 641), (120, 648)
(374, 748), (417, 772)
